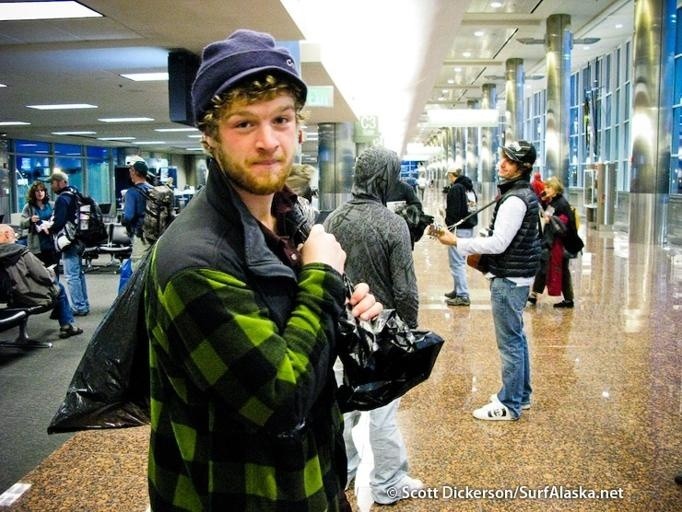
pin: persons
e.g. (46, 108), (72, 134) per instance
(18, 160), (585, 319)
(321, 143), (426, 508)
(140, 28), (384, 512)
(426, 140), (542, 421)
(1, 222), (84, 339)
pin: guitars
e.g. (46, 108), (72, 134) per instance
(427, 222), (480, 269)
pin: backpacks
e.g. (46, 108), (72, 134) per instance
(134, 186), (174, 240)
(61, 190), (107, 243)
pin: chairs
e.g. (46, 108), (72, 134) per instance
(1, 310), (53, 349)
(82, 202), (136, 272)
(0, 264), (54, 315)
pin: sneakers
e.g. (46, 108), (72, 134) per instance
(528, 296), (536, 303)
(72, 308), (87, 315)
(447, 297), (470, 306)
(490, 393), (530, 409)
(472, 401), (518, 420)
(373, 476), (422, 505)
(445, 291), (456, 298)
(554, 300), (574, 308)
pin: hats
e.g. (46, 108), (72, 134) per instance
(128, 161), (148, 173)
(191, 30), (306, 130)
(499, 140), (536, 164)
(46, 172), (67, 183)
(445, 165), (460, 176)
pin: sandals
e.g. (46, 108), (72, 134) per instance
(60, 325), (83, 339)
